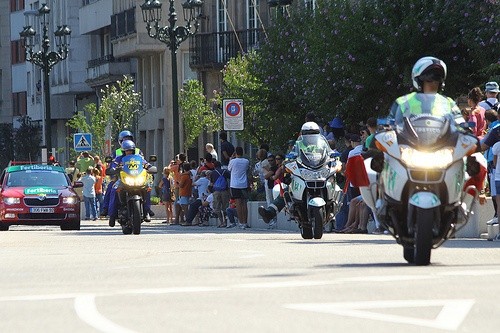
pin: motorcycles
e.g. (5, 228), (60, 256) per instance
(105, 155), (157, 235)
(367, 92), (482, 266)
(282, 134), (345, 240)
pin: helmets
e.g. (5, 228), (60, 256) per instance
(301, 122), (320, 134)
(121, 140), (136, 155)
(118, 131), (133, 145)
(411, 56), (447, 91)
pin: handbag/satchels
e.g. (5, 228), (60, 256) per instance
(268, 178), (274, 189)
(67, 168), (76, 183)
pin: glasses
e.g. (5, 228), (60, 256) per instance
(268, 160), (273, 162)
(467, 97), (470, 100)
(275, 158), (281, 160)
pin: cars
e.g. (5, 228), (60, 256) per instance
(0, 160), (84, 231)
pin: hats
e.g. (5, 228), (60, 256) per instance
(349, 134), (361, 142)
(330, 118), (343, 128)
(485, 82), (500, 92)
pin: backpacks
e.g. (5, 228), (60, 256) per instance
(213, 175), (226, 191)
(484, 98), (500, 114)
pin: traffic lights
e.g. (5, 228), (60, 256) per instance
(49, 156), (54, 165)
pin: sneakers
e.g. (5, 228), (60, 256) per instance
(487, 217), (498, 225)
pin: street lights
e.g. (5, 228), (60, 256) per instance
(139, 0), (206, 159)
(19, 3), (73, 164)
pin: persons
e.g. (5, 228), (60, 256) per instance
(369, 55), (475, 225)
(71, 150), (116, 220)
(158, 142), (250, 230)
(253, 81), (500, 233)
(100, 130), (155, 228)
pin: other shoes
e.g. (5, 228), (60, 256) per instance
(84, 218), (97, 221)
(148, 210), (154, 216)
(101, 209), (107, 216)
(162, 221), (209, 226)
(258, 206), (271, 223)
(216, 224), (228, 228)
(332, 226), (368, 234)
(109, 217), (115, 226)
(227, 223), (236, 228)
(143, 213), (150, 222)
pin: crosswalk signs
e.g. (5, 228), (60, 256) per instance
(74, 133), (92, 151)
